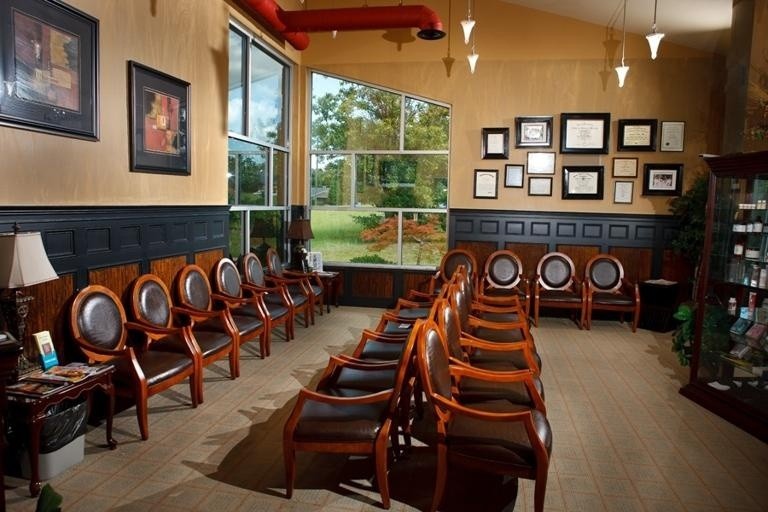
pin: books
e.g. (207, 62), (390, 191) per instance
(5, 361), (115, 401)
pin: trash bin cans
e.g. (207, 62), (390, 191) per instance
(2, 398), (88, 480)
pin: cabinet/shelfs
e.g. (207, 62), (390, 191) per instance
(672, 142), (767, 447)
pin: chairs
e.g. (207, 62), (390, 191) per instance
(68, 283), (199, 441)
(414, 321), (552, 511)
(130, 274), (238, 405)
(281, 318), (425, 511)
(265, 247), (342, 340)
(336, 261), (547, 402)
(425, 244), (644, 335)
(215, 257), (292, 358)
(174, 264), (267, 378)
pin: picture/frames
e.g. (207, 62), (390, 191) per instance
(558, 110), (612, 157)
(123, 58), (192, 180)
(307, 250), (324, 273)
(0, 0), (102, 144)
(470, 151), (686, 205)
(478, 126), (510, 162)
(513, 114), (553, 150)
(616, 118), (659, 152)
(659, 119), (686, 154)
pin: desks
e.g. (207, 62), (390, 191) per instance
(1, 354), (118, 497)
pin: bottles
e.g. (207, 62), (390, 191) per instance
(725, 172), (767, 288)
(725, 297), (737, 314)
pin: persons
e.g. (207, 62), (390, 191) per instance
(42, 343), (53, 356)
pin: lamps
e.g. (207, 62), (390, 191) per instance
(286, 215), (315, 274)
(1, 217), (63, 375)
(466, 0), (480, 76)
(610, 0), (633, 89)
(459, 0), (476, 46)
(642, 0), (665, 63)
